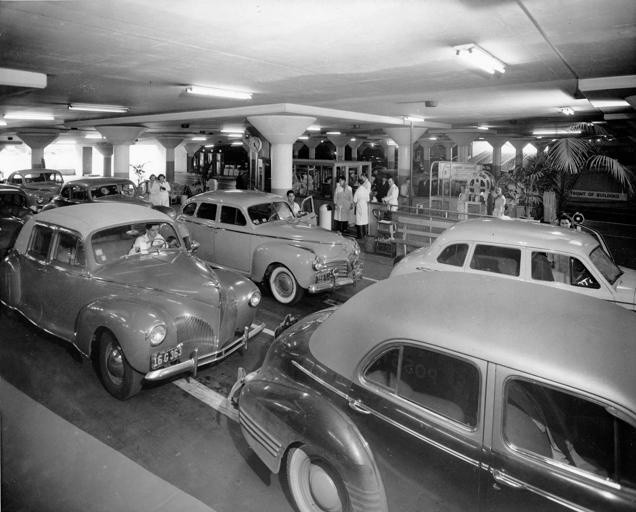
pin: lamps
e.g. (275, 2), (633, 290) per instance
(456, 43), (508, 76)
(185, 85), (253, 101)
(68, 102), (129, 112)
(0, 67), (47, 90)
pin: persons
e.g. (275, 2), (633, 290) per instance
(554, 216), (572, 274)
(148, 174), (171, 207)
(324, 169), (411, 240)
(129, 225), (169, 254)
(487, 186), (506, 217)
(278, 190), (301, 219)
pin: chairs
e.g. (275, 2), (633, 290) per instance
(374, 348), (609, 480)
(533, 254), (554, 282)
(447, 249), (499, 274)
(54, 232), (136, 270)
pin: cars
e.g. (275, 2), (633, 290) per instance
(174, 190), (364, 307)
(1, 169), (154, 255)
(0, 201), (266, 401)
(386, 216), (636, 312)
(225, 273), (635, 512)
